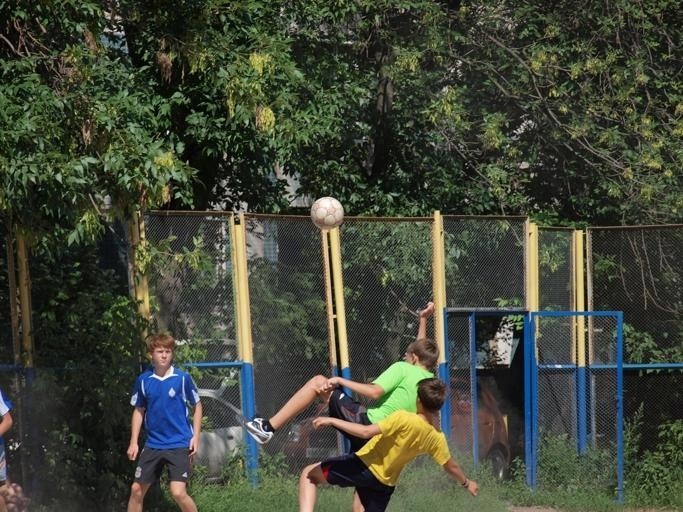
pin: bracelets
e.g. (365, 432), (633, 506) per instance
(462, 478), (469, 490)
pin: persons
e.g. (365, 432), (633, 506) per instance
(233, 302), (439, 511)
(122, 335), (204, 512)
(297, 377), (479, 511)
(0, 387), (17, 511)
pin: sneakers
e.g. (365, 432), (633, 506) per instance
(234, 414), (274, 446)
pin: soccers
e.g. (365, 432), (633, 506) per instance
(311, 196), (345, 230)
